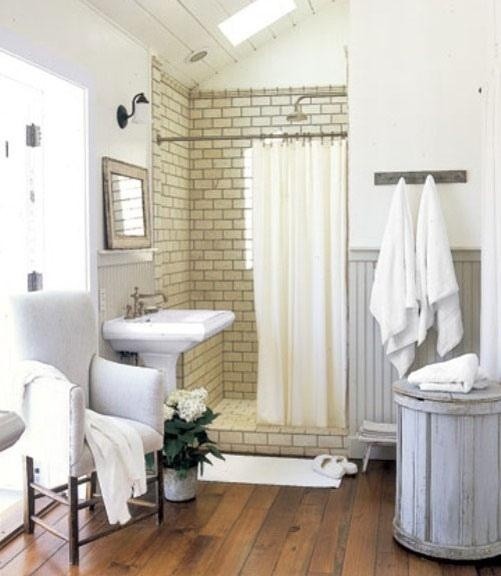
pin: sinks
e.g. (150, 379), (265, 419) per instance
(102, 309), (235, 353)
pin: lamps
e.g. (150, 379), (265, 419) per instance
(117, 92), (153, 129)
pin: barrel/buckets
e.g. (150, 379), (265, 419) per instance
(392, 381), (501, 560)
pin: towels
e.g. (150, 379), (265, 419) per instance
(368, 171), (492, 394)
(7, 359), (149, 526)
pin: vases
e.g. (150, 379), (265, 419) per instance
(161, 462), (198, 503)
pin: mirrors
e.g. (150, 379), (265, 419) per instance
(102, 155), (152, 250)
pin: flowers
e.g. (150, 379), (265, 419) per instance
(160, 385), (227, 477)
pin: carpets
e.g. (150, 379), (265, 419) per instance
(196, 452), (342, 489)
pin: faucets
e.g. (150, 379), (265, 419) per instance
(131, 287), (167, 314)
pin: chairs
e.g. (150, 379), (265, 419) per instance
(6, 290), (166, 566)
